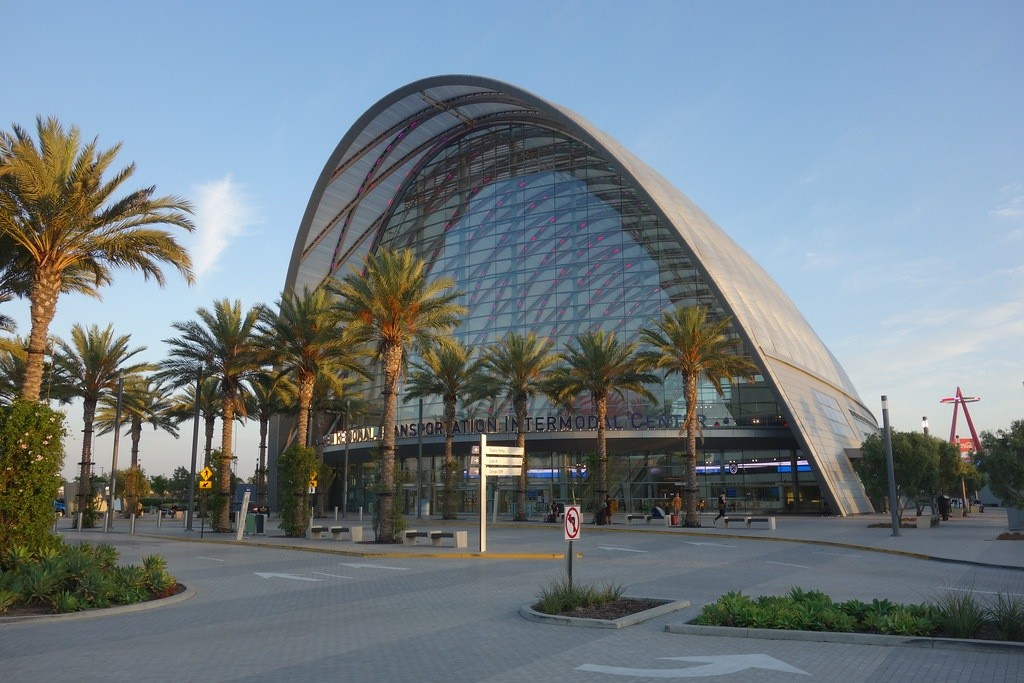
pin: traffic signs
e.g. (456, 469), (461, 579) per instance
(468, 468), (522, 478)
(472, 446), (524, 456)
(470, 457), (524, 466)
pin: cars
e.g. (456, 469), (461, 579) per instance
(53, 501), (66, 517)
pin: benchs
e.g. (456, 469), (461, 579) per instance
(723, 515), (776, 530)
(402, 529), (467, 548)
(627, 514), (671, 527)
(916, 516), (940, 529)
(309, 525), (363, 541)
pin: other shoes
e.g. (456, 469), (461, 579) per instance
(606, 523), (613, 526)
(713, 518), (716, 524)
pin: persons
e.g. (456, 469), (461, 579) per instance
(713, 492), (726, 524)
(606, 494), (612, 524)
(672, 492), (682, 516)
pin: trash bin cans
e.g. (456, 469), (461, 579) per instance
(246, 513), (267, 535)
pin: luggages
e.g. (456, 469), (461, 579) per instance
(672, 514), (678, 525)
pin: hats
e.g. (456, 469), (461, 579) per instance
(607, 494), (610, 498)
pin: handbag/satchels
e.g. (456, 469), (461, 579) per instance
(600, 501), (607, 509)
(671, 499), (674, 507)
